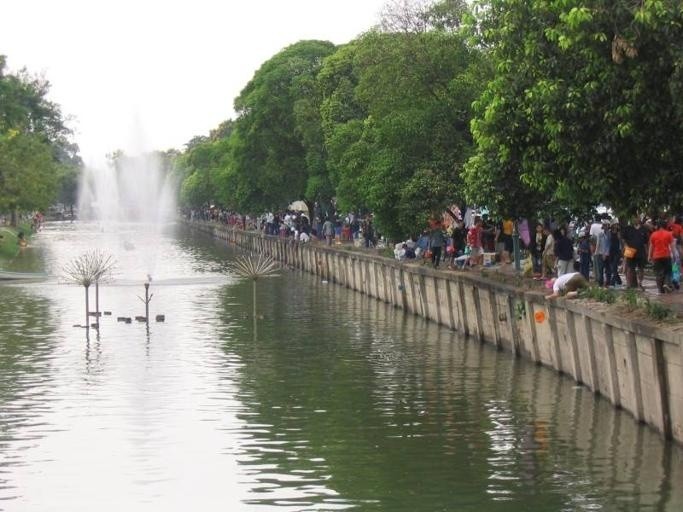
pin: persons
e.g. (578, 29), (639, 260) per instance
(16, 208), (45, 247)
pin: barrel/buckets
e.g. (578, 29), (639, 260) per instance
(535, 310), (545, 323)
